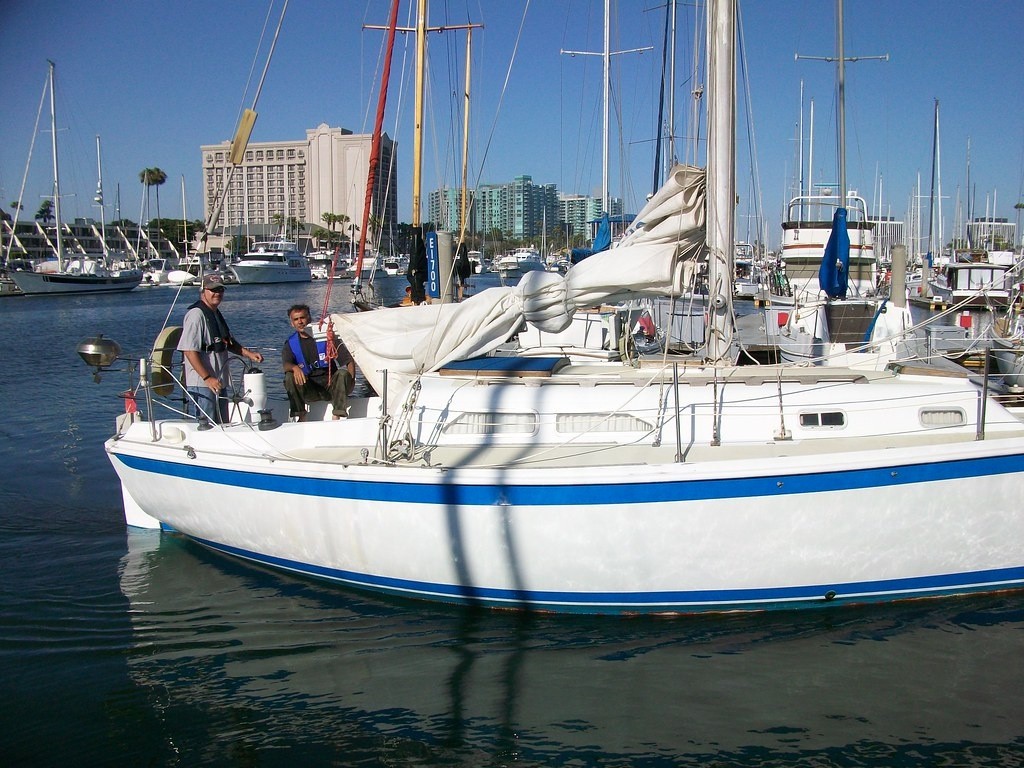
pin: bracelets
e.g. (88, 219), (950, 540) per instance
(203, 375), (211, 380)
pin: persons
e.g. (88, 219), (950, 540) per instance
(177, 275), (264, 425)
(282, 306), (355, 421)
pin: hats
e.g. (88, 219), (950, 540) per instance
(204, 274), (227, 290)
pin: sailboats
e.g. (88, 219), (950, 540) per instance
(1, 0), (1024, 616)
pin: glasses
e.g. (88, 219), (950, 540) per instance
(210, 287), (225, 292)
